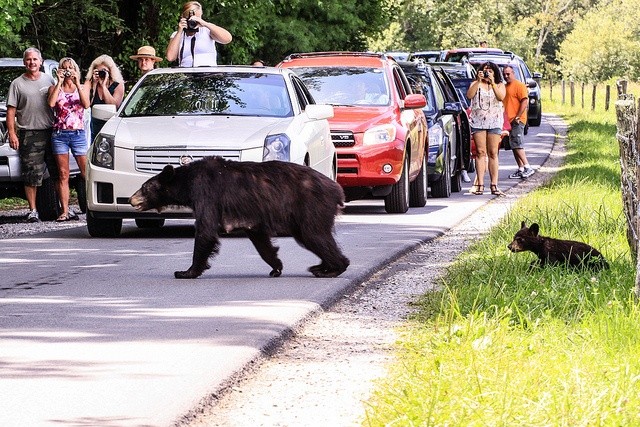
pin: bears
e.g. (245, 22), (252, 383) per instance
(129, 154), (350, 277)
(507, 220), (610, 276)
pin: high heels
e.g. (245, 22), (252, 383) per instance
(460, 170), (471, 183)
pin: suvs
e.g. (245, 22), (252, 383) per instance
(406, 50), (445, 60)
(446, 48), (506, 61)
(461, 49), (543, 126)
(384, 51), (410, 61)
(275, 50), (429, 212)
(0, 55), (91, 218)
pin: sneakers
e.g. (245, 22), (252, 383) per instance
(521, 167), (536, 178)
(58, 206), (80, 220)
(508, 169), (522, 179)
(26, 208), (39, 222)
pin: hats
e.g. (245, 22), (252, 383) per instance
(129, 46), (164, 62)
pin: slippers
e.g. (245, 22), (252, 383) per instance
(55, 212), (70, 222)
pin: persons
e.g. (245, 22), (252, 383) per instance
(166, 1), (233, 66)
(130, 46), (162, 75)
(84, 55), (124, 144)
(466, 61), (506, 196)
(6, 49), (78, 222)
(48, 58), (88, 222)
(502, 65), (535, 179)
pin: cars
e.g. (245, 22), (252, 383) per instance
(83, 64), (337, 236)
(395, 57), (471, 197)
(431, 60), (477, 112)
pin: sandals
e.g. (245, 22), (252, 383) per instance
(475, 185), (484, 195)
(489, 184), (501, 195)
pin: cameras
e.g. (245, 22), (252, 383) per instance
(95, 70), (106, 79)
(61, 70), (71, 79)
(482, 70), (489, 79)
(183, 11), (199, 32)
